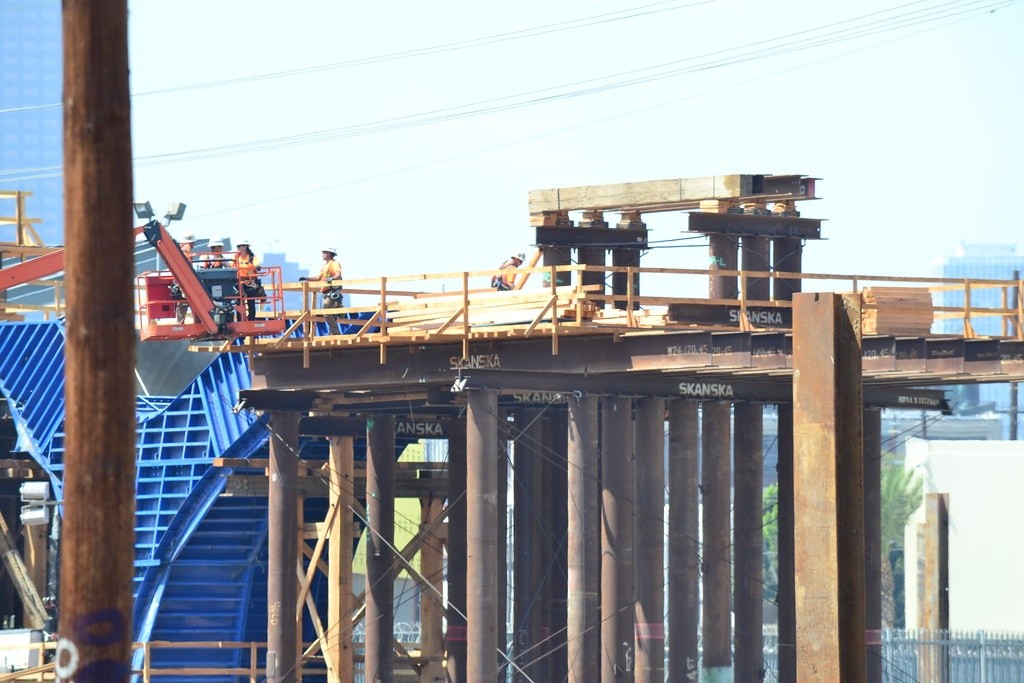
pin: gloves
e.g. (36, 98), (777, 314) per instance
(256, 265), (261, 271)
(299, 277), (307, 282)
(327, 279), (333, 284)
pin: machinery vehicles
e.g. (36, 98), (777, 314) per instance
(2, 201), (288, 347)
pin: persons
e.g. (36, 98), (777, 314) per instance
(496, 250), (526, 291)
(231, 237), (263, 321)
(198, 234), (235, 270)
(298, 244), (345, 336)
(168, 234), (198, 324)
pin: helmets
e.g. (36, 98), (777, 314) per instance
(178, 232), (197, 243)
(207, 237), (224, 247)
(321, 245), (339, 256)
(510, 250), (525, 262)
(234, 238), (251, 246)
